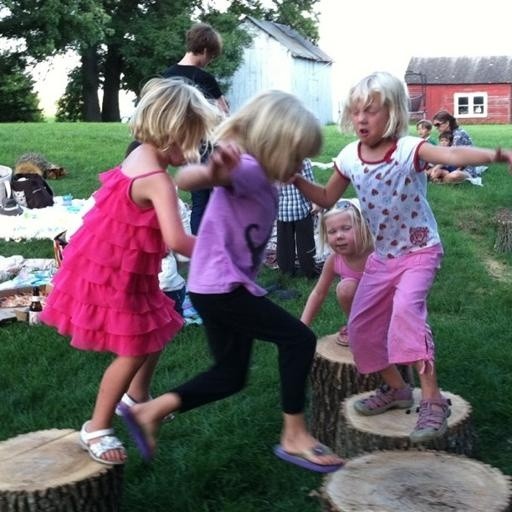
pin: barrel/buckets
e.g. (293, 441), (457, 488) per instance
(272, 437), (341, 474)
(118, 401), (157, 465)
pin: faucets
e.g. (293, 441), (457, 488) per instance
(78, 419), (127, 465)
(409, 393), (452, 444)
(354, 379), (413, 415)
(335, 325), (350, 346)
(114, 393), (175, 423)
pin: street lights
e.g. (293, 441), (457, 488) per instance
(11, 173), (54, 209)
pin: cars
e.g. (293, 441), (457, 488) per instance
(0, 164), (13, 210)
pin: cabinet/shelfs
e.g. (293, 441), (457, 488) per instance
(30, 285), (43, 327)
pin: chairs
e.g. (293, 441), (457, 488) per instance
(306, 261), (326, 279)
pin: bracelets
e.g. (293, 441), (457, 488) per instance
(493, 148), (502, 161)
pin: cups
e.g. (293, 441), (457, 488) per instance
(324, 201), (360, 218)
(433, 121), (447, 128)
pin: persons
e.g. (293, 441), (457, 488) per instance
(431, 110), (477, 184)
(300, 198), (375, 347)
(279, 71), (509, 443)
(114, 90), (345, 472)
(276, 157), (324, 280)
(39, 76), (224, 467)
(427, 132), (455, 183)
(416, 119), (438, 146)
(160, 25), (230, 238)
